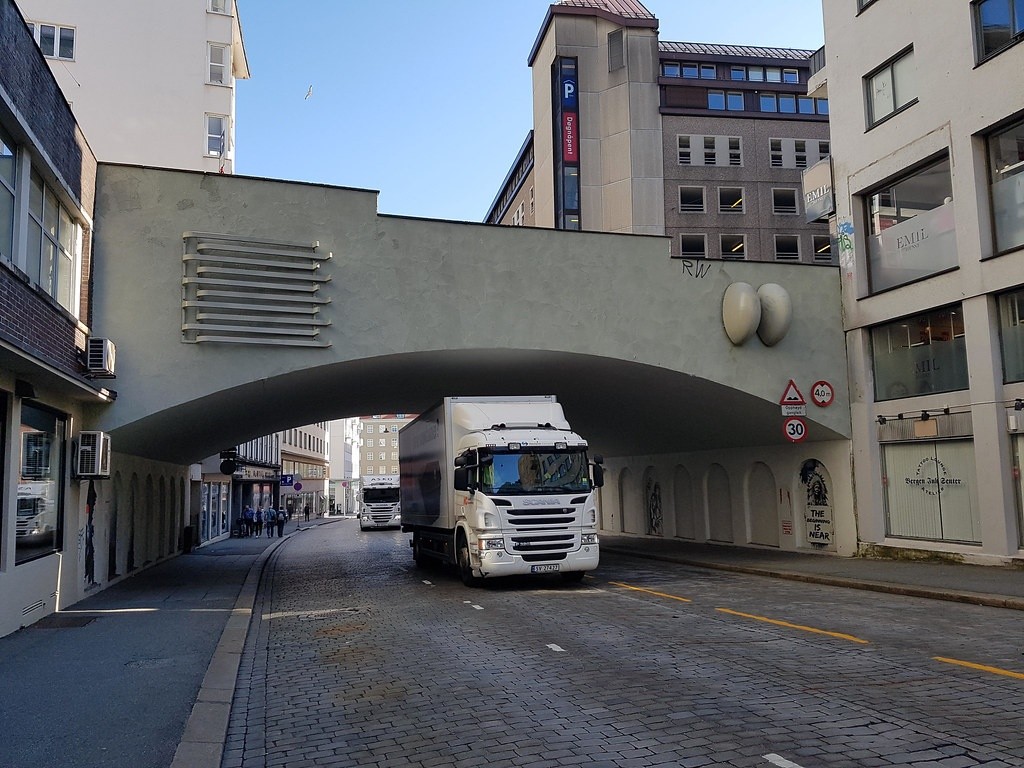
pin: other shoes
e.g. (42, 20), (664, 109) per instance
(259, 535), (261, 537)
(256, 536), (258, 538)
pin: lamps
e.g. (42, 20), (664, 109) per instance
(874, 407), (950, 425)
(1014, 397), (1024, 411)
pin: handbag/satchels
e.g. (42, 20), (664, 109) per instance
(253, 514), (257, 522)
(271, 516), (275, 520)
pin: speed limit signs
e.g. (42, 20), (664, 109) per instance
(785, 417), (806, 443)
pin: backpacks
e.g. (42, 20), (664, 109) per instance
(278, 513), (284, 520)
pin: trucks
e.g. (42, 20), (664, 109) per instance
(355, 474), (400, 532)
(400, 394), (610, 589)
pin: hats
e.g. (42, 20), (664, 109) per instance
(269, 506), (272, 508)
(246, 506), (249, 508)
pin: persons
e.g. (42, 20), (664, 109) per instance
(287, 504), (294, 521)
(240, 504), (288, 539)
(303, 503), (310, 522)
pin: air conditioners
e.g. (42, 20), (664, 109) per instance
(87, 337), (117, 375)
(21, 432), (51, 477)
(77, 431), (112, 476)
